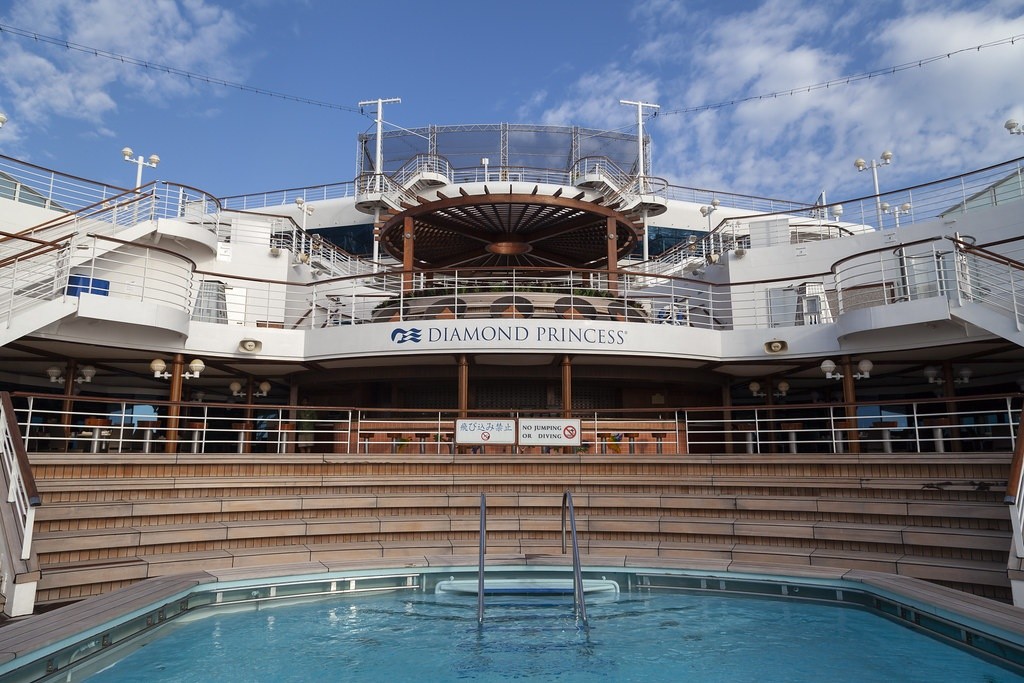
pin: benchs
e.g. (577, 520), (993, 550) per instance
(27, 448), (1018, 593)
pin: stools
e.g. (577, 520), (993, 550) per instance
(360, 431), (667, 455)
